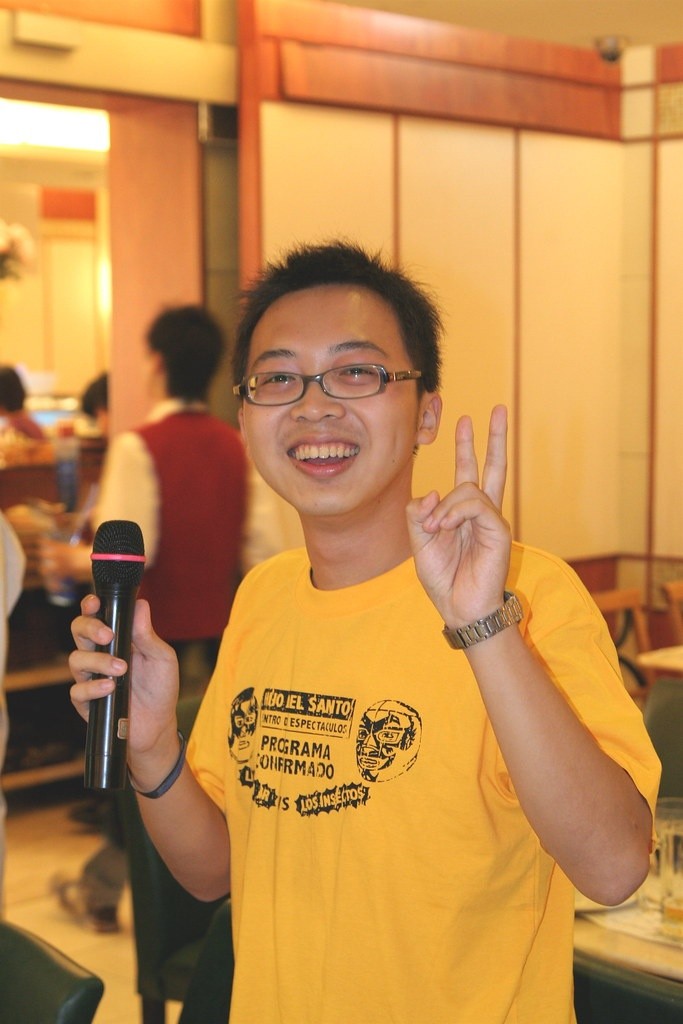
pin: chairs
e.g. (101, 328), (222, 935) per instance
(591, 580), (683, 699)
(0, 898), (234, 1024)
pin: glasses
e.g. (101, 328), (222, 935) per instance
(232, 363), (422, 406)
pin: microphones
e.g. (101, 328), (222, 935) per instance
(83, 520), (147, 789)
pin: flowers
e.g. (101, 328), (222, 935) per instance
(0, 219), (37, 283)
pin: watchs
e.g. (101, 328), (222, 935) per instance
(442, 590), (524, 650)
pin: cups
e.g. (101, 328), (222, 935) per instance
(638, 795), (683, 937)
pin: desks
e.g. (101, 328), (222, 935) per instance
(575, 818), (683, 1024)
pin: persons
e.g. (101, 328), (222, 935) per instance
(70, 237), (662, 1023)
(0, 366), (108, 827)
(50, 301), (283, 933)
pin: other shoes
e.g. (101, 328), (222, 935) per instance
(71, 798), (106, 823)
(52, 868), (118, 931)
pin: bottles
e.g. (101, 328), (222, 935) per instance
(55, 425), (80, 510)
(38, 505), (78, 607)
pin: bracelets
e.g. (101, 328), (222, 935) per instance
(127, 730), (186, 799)
